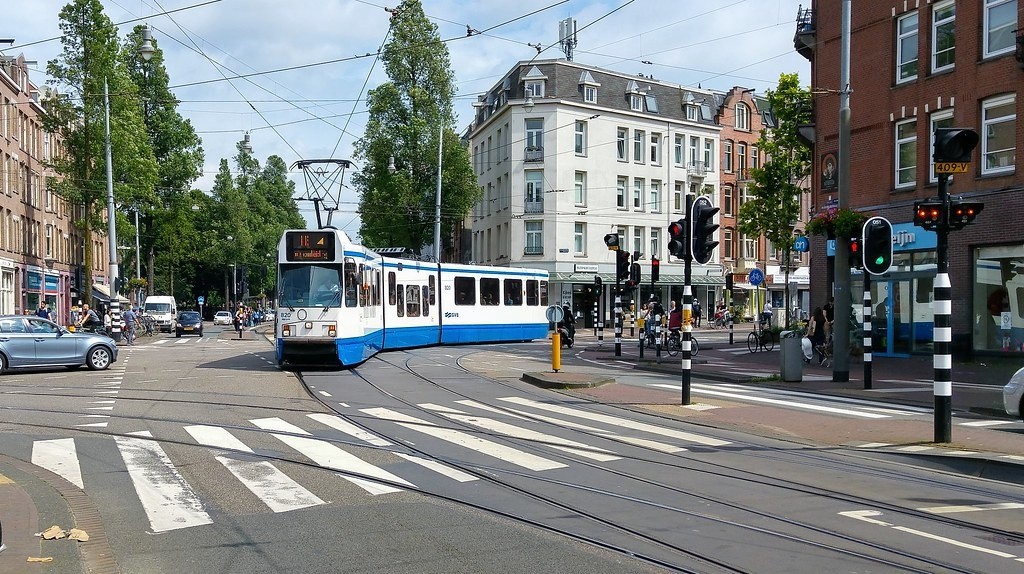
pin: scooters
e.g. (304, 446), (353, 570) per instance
(557, 319), (578, 348)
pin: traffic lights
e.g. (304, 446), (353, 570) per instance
(619, 250), (631, 279)
(667, 218), (688, 260)
(861, 215), (894, 277)
(652, 259), (660, 282)
(595, 277), (602, 298)
(691, 195), (720, 267)
(947, 201), (985, 229)
(603, 233), (619, 251)
(934, 127), (981, 163)
(914, 198), (942, 231)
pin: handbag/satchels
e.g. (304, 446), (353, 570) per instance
(801, 337), (813, 359)
(807, 316), (816, 336)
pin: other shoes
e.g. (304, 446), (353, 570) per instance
(568, 338), (572, 341)
(821, 357), (827, 365)
(802, 359), (809, 364)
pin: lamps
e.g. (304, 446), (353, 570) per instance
(588, 288), (591, 292)
(140, 29), (156, 61)
(523, 89), (536, 113)
(387, 156), (396, 173)
(243, 135), (251, 153)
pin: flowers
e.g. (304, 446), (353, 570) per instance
(130, 277), (148, 288)
(806, 207), (878, 237)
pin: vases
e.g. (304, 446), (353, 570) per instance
(824, 229), (836, 240)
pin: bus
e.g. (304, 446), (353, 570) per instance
(271, 158), (551, 374)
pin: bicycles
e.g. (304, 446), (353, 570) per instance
(708, 309), (730, 330)
(666, 326), (700, 357)
(747, 319), (774, 354)
(637, 318), (663, 351)
(69, 323), (110, 338)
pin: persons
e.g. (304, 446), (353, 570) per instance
(34, 301), (142, 347)
(316, 276), (514, 317)
(876, 297), (889, 320)
(234, 304), (271, 332)
(559, 297), (833, 368)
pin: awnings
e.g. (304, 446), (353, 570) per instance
(75, 283), (130, 304)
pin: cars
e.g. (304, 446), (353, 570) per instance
(0, 314), (119, 375)
(174, 311), (206, 338)
(213, 311), (232, 325)
(261, 310), (275, 322)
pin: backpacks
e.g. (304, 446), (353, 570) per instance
(652, 303), (665, 316)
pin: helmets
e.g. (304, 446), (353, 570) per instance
(563, 304), (569, 309)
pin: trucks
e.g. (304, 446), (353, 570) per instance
(142, 296), (177, 335)
